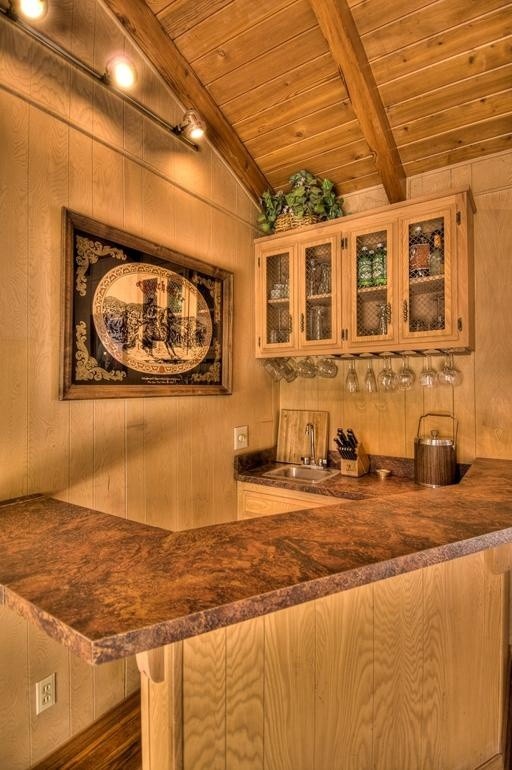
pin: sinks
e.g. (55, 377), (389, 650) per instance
(262, 463), (340, 484)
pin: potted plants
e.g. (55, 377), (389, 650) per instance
(255, 166), (346, 236)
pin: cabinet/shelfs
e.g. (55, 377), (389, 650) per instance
(342, 183), (476, 351)
(255, 216), (345, 357)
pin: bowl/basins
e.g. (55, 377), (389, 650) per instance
(270, 284), (288, 298)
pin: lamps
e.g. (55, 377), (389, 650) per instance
(0, 0), (212, 156)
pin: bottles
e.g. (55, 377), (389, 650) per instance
(358, 227), (445, 287)
(308, 259), (330, 294)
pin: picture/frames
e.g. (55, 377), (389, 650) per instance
(56, 204), (237, 400)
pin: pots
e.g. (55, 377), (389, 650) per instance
(306, 305), (328, 340)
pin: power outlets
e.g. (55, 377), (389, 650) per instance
(34, 672), (57, 714)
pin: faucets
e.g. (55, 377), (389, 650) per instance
(305, 423), (315, 457)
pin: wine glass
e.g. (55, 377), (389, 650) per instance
(344, 353), (461, 394)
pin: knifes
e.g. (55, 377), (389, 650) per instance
(335, 427), (357, 460)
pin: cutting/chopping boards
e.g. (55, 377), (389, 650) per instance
(276, 409), (329, 465)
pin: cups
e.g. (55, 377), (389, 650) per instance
(263, 357), (339, 383)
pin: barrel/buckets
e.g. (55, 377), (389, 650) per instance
(414, 415), (457, 487)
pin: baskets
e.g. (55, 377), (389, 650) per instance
(274, 213), (324, 233)
